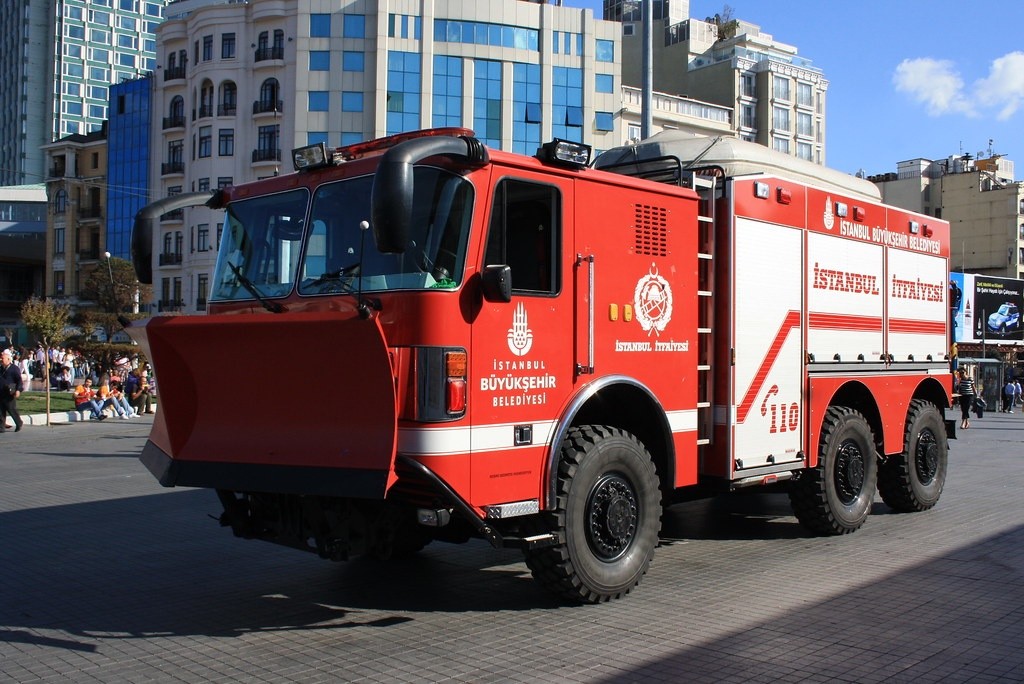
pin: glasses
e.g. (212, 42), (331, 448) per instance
(959, 372), (964, 374)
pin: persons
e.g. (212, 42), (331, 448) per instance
(0, 354), (24, 433)
(1001, 379), (1024, 414)
(956, 368), (977, 429)
(2, 345), (154, 422)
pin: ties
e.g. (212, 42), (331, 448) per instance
(2, 367), (7, 375)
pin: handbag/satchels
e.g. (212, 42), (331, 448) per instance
(971, 398), (986, 418)
(8, 383), (18, 395)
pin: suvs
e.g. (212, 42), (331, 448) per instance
(988, 302), (1022, 336)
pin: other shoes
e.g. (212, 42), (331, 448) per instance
(146, 411), (154, 414)
(5, 425), (12, 428)
(31, 378), (35, 381)
(129, 413), (141, 418)
(1003, 411), (1007, 413)
(90, 416), (98, 419)
(137, 412), (144, 415)
(15, 421), (23, 431)
(41, 378), (45, 382)
(960, 423), (969, 429)
(120, 414), (129, 420)
(1009, 411), (1014, 414)
(98, 415), (107, 421)
(0, 428), (4, 433)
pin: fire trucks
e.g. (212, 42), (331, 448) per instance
(140, 128), (963, 608)
